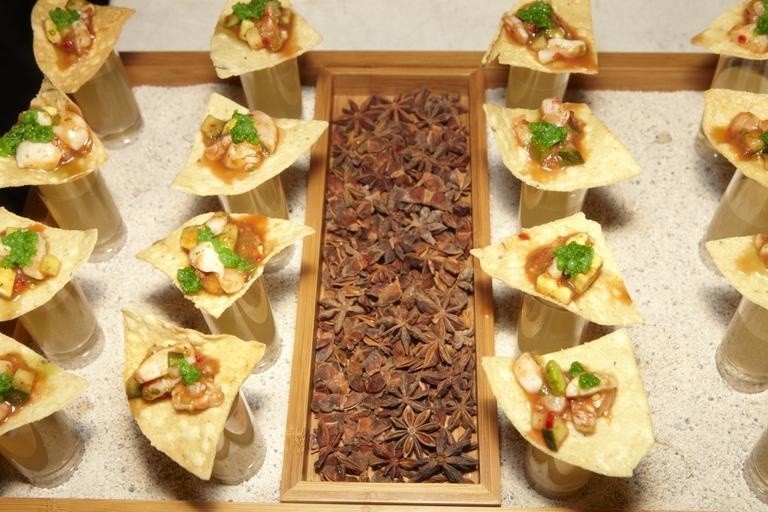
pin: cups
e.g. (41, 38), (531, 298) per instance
(516, 288), (585, 358)
(694, 52), (768, 165)
(240, 53), (302, 120)
(17, 270), (105, 371)
(742, 424), (768, 506)
(201, 268), (281, 374)
(518, 181), (588, 235)
(217, 166), (294, 272)
(525, 440), (592, 499)
(38, 163), (128, 262)
(506, 57), (570, 109)
(0, 392), (85, 489)
(698, 166), (768, 240)
(208, 383), (267, 484)
(715, 295), (768, 394)
(72, 47), (146, 151)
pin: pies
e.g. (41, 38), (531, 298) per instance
(29, 1), (136, 95)
(168, 92), (329, 197)
(479, 328), (654, 477)
(210, 0), (322, 79)
(470, 212), (645, 328)
(701, 87), (768, 189)
(482, 101), (641, 190)
(0, 332), (89, 437)
(479, 1), (600, 75)
(136, 211), (315, 319)
(0, 206), (97, 322)
(121, 307), (267, 481)
(689, 0), (768, 61)
(705, 233), (768, 311)
(0, 75), (110, 191)
